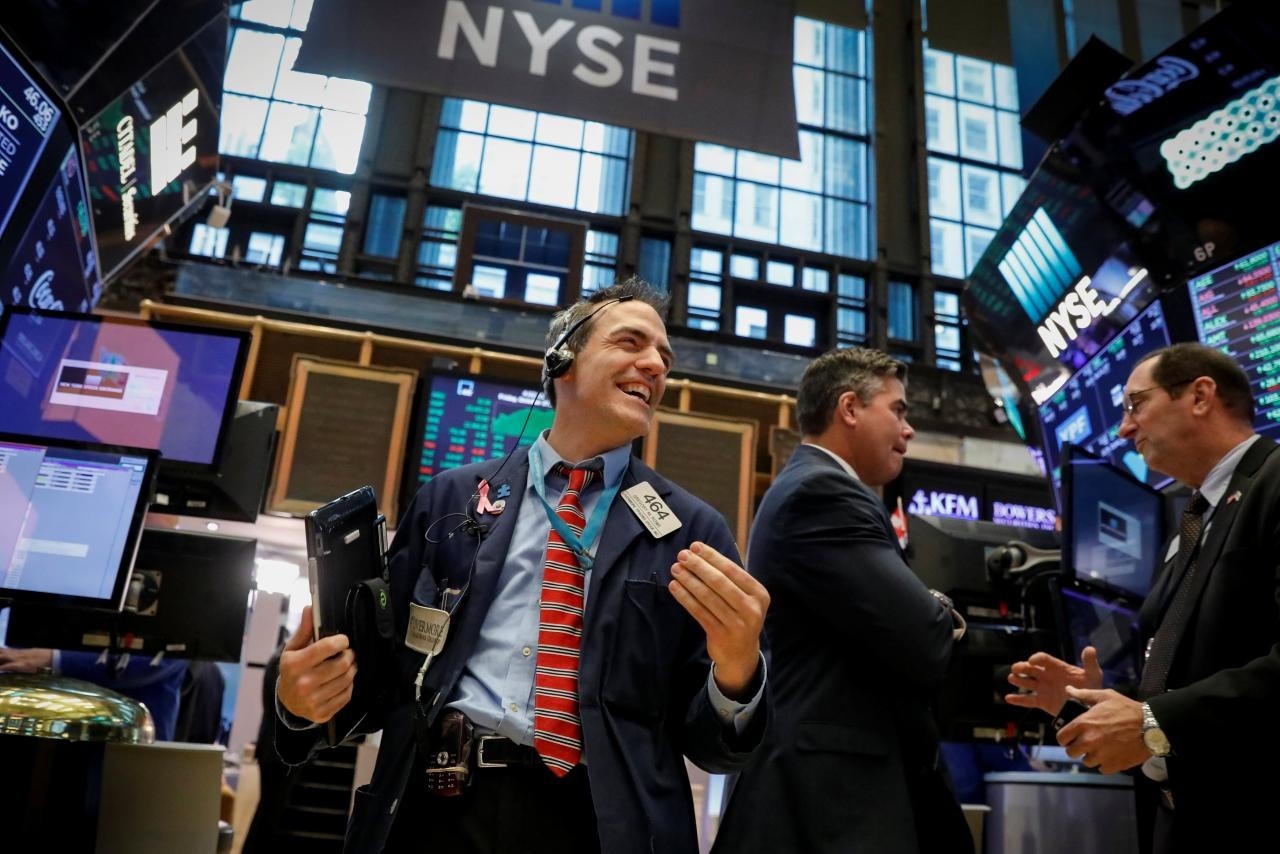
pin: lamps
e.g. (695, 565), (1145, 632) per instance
(204, 182), (235, 227)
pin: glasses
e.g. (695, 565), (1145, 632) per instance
(1123, 383), (1173, 415)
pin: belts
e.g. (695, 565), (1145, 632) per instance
(470, 734), (540, 770)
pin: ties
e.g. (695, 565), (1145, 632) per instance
(1136, 490), (1211, 700)
(533, 462), (605, 778)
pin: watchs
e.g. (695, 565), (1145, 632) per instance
(928, 588), (955, 614)
(1141, 700), (1170, 756)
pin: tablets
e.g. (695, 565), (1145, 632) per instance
(307, 486), (393, 749)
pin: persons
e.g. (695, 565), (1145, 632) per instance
(1004, 344), (1280, 854)
(0, 602), (332, 854)
(261, 275), (778, 854)
(700, 345), (979, 854)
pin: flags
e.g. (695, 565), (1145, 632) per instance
(890, 506), (908, 549)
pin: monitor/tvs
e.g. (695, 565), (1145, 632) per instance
(905, 511), (1063, 631)
(2, 526), (256, 662)
(0, 307), (252, 477)
(1061, 447), (1165, 612)
(0, 433), (162, 612)
(149, 400), (278, 522)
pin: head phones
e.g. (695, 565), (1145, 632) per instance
(544, 294), (636, 376)
